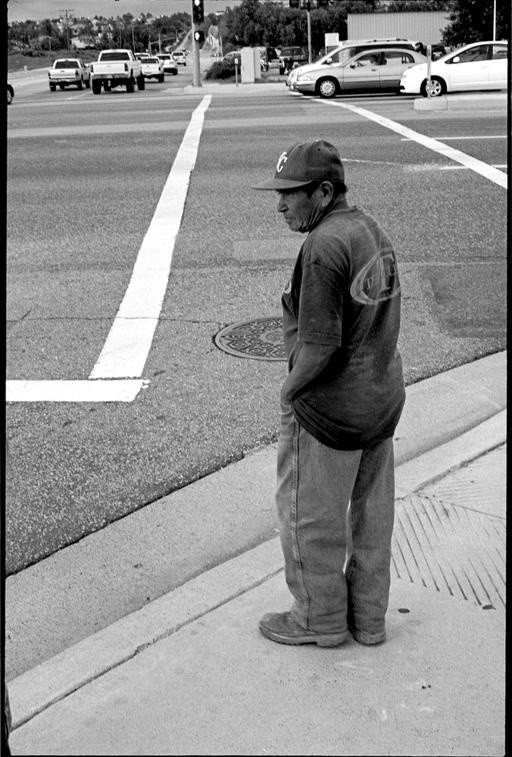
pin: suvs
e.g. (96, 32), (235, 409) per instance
(137, 54), (166, 82)
(284, 37), (419, 94)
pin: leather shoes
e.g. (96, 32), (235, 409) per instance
(348, 623), (387, 645)
(259, 612), (351, 647)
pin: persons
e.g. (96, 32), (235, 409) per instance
(251, 139), (404, 649)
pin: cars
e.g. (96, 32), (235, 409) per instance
(6, 82), (15, 105)
(226, 52), (241, 59)
(291, 47), (436, 99)
(131, 46), (189, 74)
(399, 38), (508, 99)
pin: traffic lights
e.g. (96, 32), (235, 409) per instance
(192, 0), (205, 24)
(194, 30), (204, 41)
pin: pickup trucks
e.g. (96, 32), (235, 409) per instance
(87, 47), (146, 94)
(46, 56), (91, 90)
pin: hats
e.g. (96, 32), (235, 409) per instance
(252, 139), (344, 191)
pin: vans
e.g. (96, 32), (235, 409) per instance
(278, 44), (307, 76)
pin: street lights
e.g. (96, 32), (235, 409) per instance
(288, 0), (335, 63)
